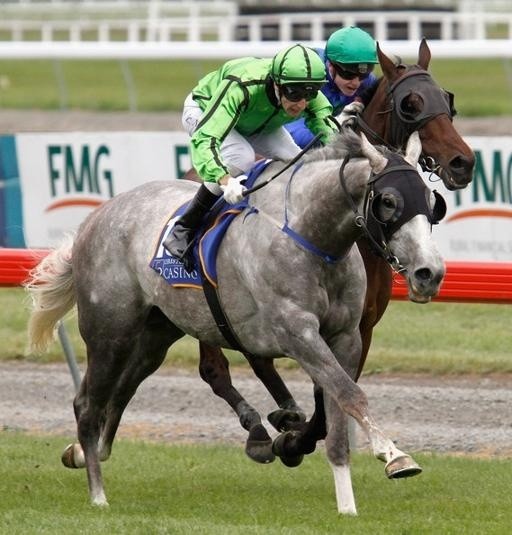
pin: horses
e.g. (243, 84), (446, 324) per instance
(18, 126), (448, 519)
(198, 37), (476, 466)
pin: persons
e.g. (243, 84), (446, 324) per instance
(158, 43), (335, 278)
(282, 24), (388, 157)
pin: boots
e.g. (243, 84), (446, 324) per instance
(163, 184), (224, 273)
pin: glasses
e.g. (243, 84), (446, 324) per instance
(281, 85), (317, 102)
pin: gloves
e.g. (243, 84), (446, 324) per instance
(334, 101), (365, 126)
(220, 178), (247, 205)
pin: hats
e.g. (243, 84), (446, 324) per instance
(269, 26), (380, 84)
